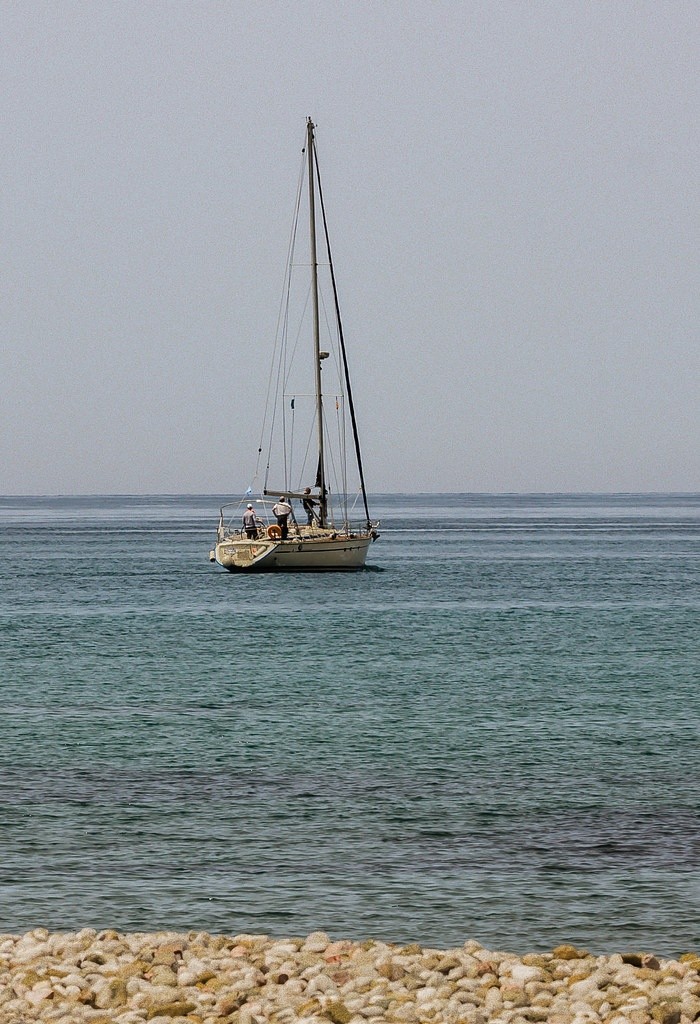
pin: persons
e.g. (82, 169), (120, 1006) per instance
(303, 487), (322, 525)
(271, 496), (292, 540)
(242, 503), (259, 540)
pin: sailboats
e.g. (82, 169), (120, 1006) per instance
(210, 116), (380, 573)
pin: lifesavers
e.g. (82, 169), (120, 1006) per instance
(267, 525), (282, 539)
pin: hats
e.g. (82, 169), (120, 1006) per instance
(247, 504), (252, 509)
(305, 487), (311, 493)
(279, 496), (284, 500)
